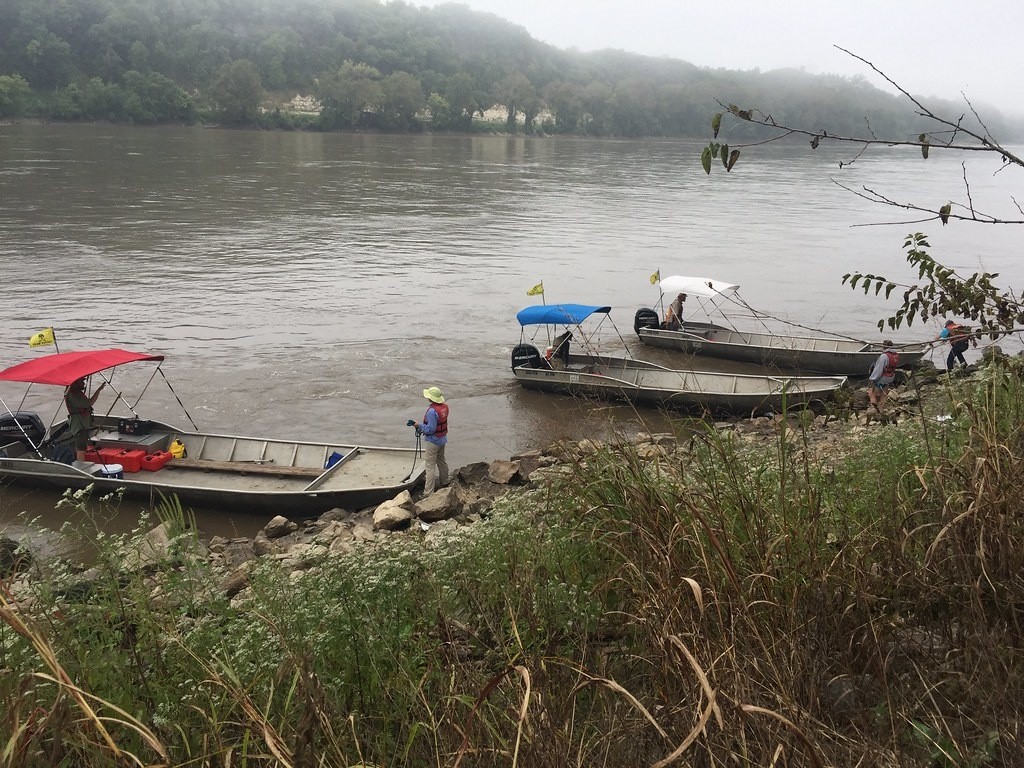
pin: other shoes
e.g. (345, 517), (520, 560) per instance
(441, 481), (450, 485)
(417, 494), (434, 500)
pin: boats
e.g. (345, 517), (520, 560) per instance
(512, 278), (849, 417)
(635, 268), (934, 377)
(0, 326), (427, 513)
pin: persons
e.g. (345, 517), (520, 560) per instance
(64, 377), (106, 461)
(869, 339), (899, 402)
(414, 387), (449, 499)
(666, 293), (688, 331)
(553, 331), (573, 368)
(930, 319), (969, 382)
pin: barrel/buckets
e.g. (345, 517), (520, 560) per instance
(101, 464), (124, 480)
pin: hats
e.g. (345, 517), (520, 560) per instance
(564, 330), (572, 339)
(678, 293), (687, 297)
(423, 387), (445, 404)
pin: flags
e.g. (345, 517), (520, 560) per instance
(650, 270), (660, 284)
(527, 283), (544, 296)
(29, 328), (54, 348)
